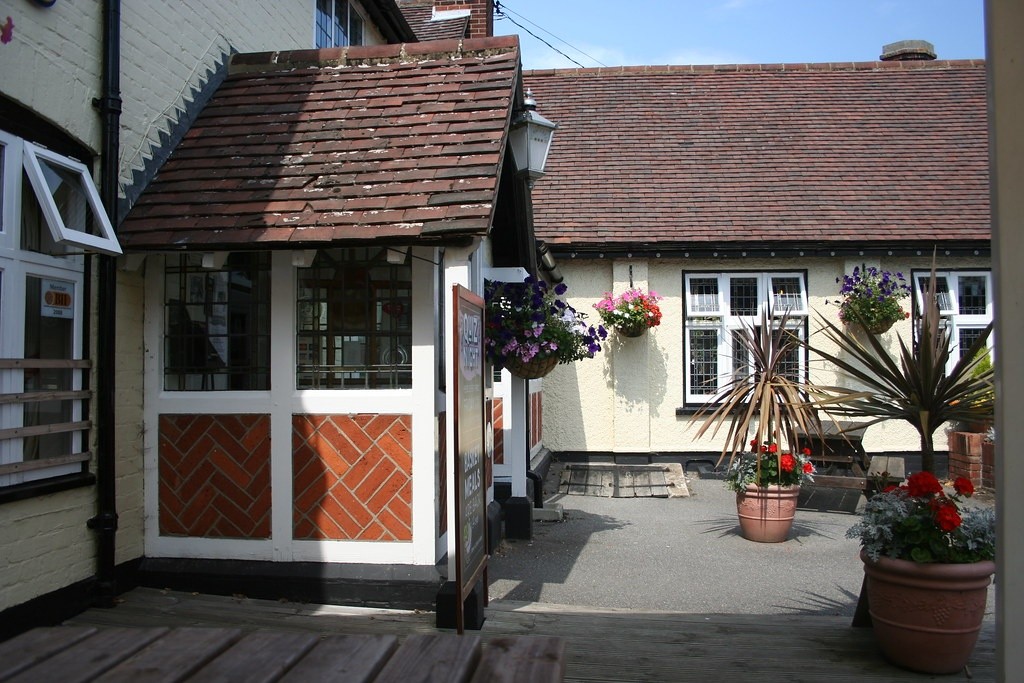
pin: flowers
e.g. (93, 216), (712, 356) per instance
(721, 430), (817, 494)
(845, 472), (995, 563)
(592, 288), (663, 330)
(822, 264), (910, 327)
(484, 277), (601, 365)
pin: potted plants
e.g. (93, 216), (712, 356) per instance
(957, 347), (999, 431)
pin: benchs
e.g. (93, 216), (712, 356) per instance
(868, 455), (905, 491)
(802, 454), (875, 503)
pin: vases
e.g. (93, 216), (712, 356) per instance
(868, 319), (896, 335)
(498, 352), (559, 379)
(860, 546), (996, 674)
(616, 323), (645, 338)
(736, 482), (802, 542)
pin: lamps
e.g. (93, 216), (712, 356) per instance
(508, 86), (558, 191)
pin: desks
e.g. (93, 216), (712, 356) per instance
(792, 420), (876, 503)
